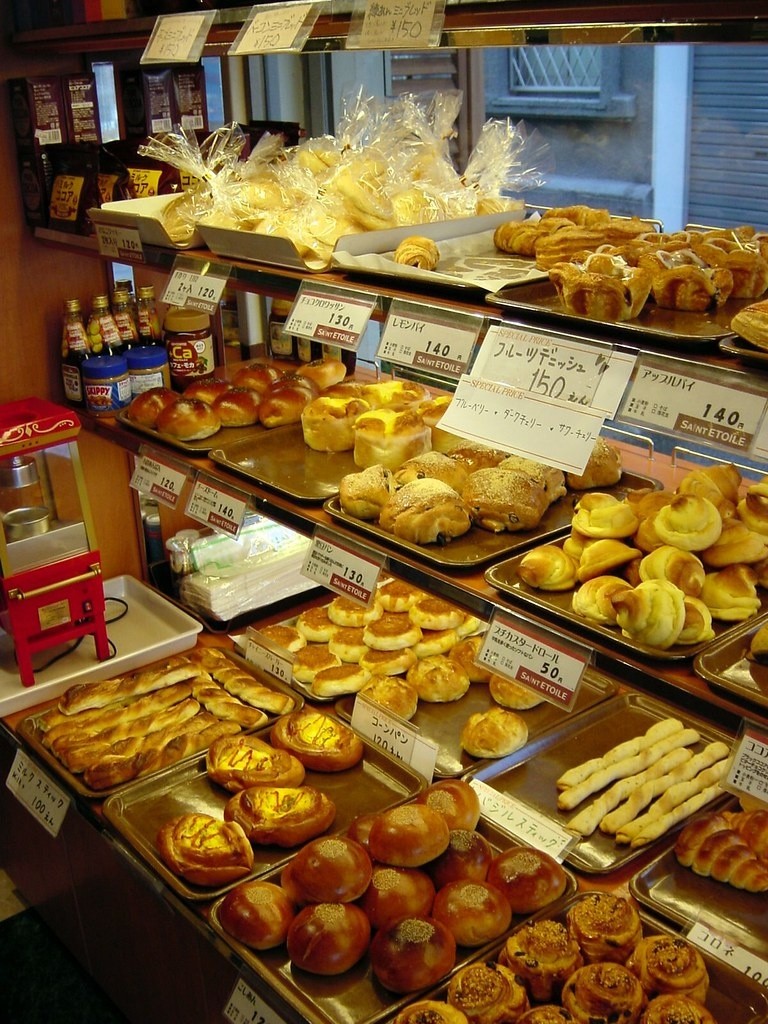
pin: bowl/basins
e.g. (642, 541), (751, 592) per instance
(2, 506), (49, 542)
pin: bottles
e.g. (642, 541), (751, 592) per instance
(268, 298), (356, 376)
(62, 280), (161, 408)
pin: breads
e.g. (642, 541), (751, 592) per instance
(30, 583), (768, 1024)
(122, 357), (768, 667)
(393, 206), (768, 353)
(163, 148), (518, 257)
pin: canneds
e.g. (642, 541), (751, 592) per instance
(83, 309), (214, 417)
